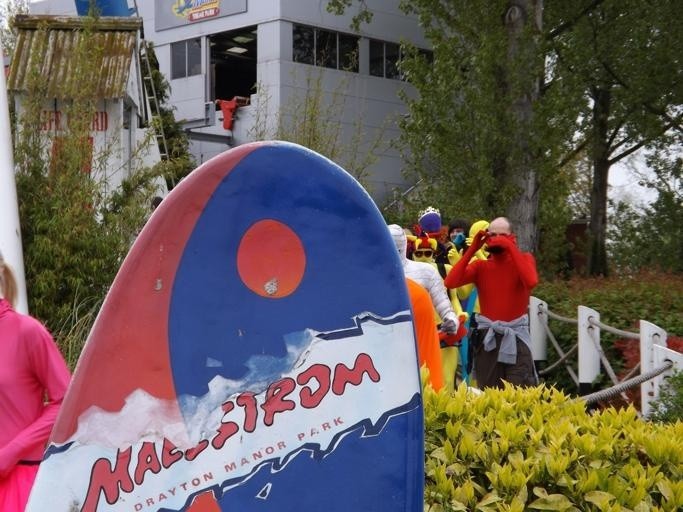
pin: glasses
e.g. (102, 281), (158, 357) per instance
(488, 232), (513, 238)
(414, 251), (433, 258)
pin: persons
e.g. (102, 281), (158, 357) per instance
(0, 251), (71, 512)
(387, 206), (540, 388)
